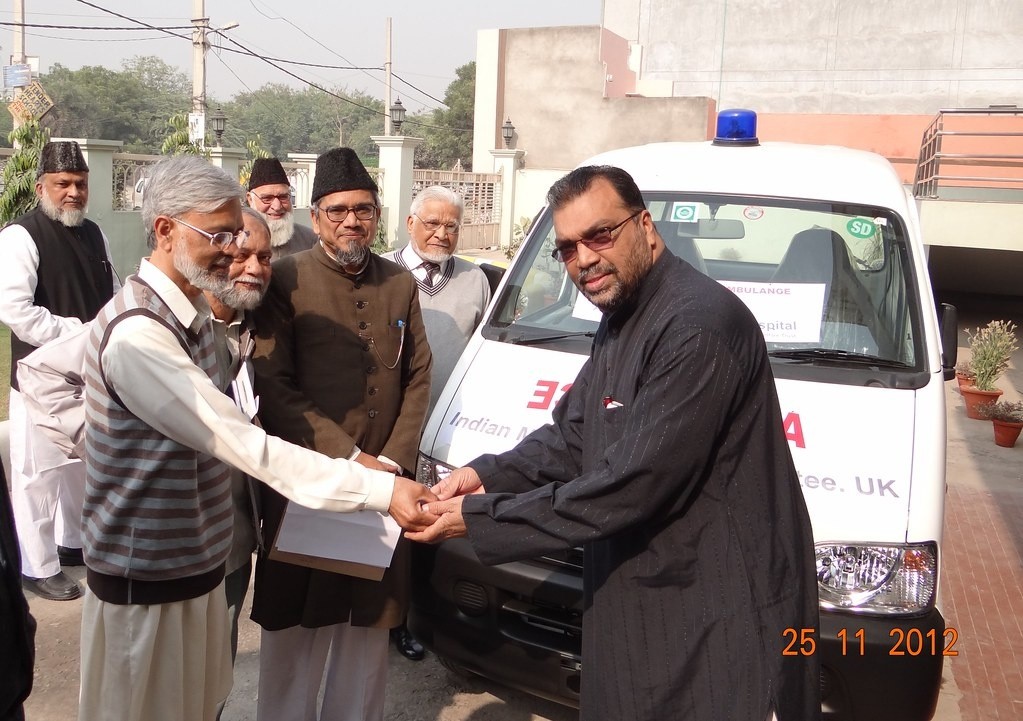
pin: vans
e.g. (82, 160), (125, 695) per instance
(411, 109), (958, 721)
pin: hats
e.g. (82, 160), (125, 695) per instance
(311, 147), (378, 205)
(37, 141), (89, 181)
(249, 157), (290, 191)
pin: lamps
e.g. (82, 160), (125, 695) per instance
(208, 103), (228, 148)
(389, 96), (407, 135)
(500, 117), (516, 150)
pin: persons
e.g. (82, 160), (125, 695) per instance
(77, 151), (443, 721)
(17, 206), (277, 721)
(0, 139), (120, 603)
(403, 166), (819, 721)
(242, 157), (318, 264)
(1, 457), (37, 720)
(375, 185), (492, 661)
(239, 147), (434, 721)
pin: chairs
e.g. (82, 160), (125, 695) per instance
(766, 228), (897, 360)
(653, 220), (708, 274)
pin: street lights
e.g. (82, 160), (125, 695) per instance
(193, 22), (240, 146)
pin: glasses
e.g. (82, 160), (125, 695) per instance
(172, 218), (250, 251)
(316, 202), (378, 222)
(413, 214), (463, 235)
(552, 211), (642, 261)
(252, 191), (291, 204)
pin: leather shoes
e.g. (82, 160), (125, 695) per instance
(390, 624), (425, 660)
(22, 571), (81, 600)
(57, 545), (87, 567)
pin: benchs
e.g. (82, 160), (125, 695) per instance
(705, 259), (885, 317)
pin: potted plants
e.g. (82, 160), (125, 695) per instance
(953, 320), (1023, 448)
